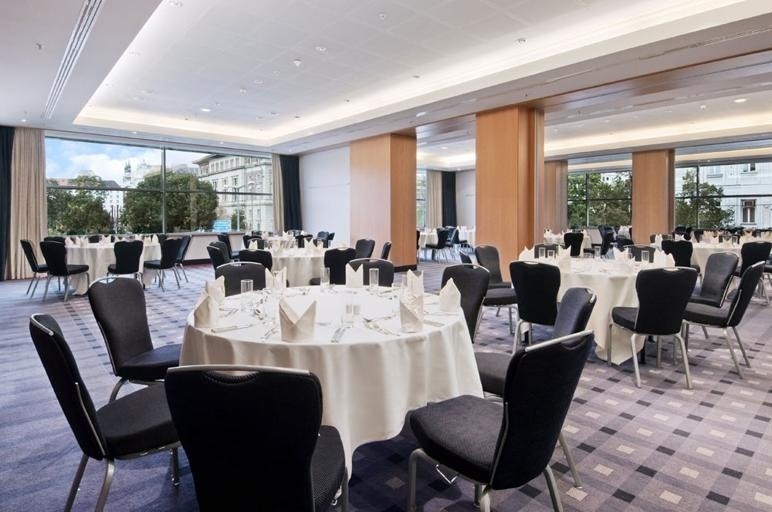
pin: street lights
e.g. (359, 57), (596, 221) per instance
(222, 180), (256, 231)
(587, 205), (597, 226)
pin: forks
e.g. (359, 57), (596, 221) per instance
(330, 315), (353, 343)
(362, 317), (400, 336)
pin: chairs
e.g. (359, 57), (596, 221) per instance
(475, 286), (595, 490)
(404, 330), (596, 512)
(475, 225), (772, 388)
(29, 312), (183, 511)
(20, 230), (198, 304)
(206, 229), (396, 301)
(440, 265), (492, 343)
(415, 225), (478, 271)
(163, 364), (349, 511)
(87, 275), (184, 488)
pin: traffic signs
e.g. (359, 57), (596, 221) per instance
(211, 218), (231, 232)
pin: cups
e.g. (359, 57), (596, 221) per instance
(264, 230), (322, 257)
(241, 280), (253, 310)
(369, 268), (379, 293)
(82, 232), (135, 247)
(539, 246), (649, 270)
(262, 288), (273, 320)
(700, 236), (737, 249)
(392, 282), (401, 312)
(321, 267), (330, 293)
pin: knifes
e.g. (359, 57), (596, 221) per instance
(226, 306), (239, 317)
(211, 322), (252, 333)
(262, 323), (279, 342)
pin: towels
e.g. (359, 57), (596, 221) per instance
(277, 296), (317, 343)
(406, 269), (425, 291)
(437, 277), (463, 315)
(191, 288), (219, 329)
(400, 291), (425, 333)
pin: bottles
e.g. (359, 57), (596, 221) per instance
(345, 302), (360, 315)
